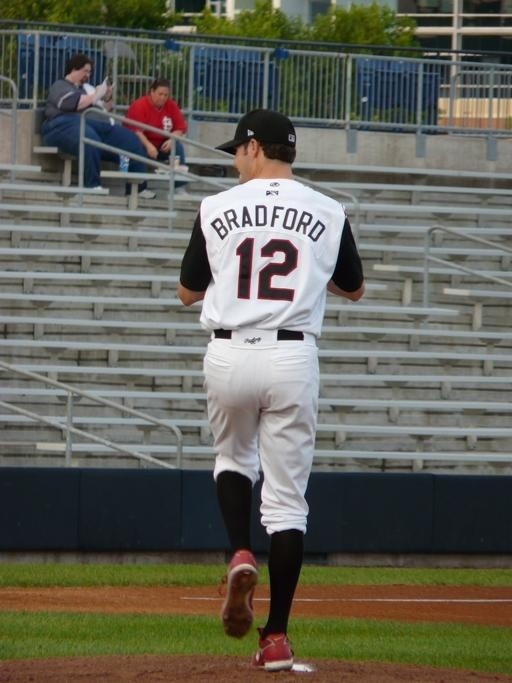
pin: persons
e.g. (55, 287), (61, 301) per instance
(175, 109), (366, 670)
(39, 53), (188, 199)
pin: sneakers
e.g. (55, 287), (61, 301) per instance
(249, 627), (293, 672)
(174, 186), (189, 195)
(124, 189), (157, 199)
(222, 549), (258, 638)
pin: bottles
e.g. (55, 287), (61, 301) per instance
(118, 152), (130, 178)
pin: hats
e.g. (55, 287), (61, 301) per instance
(215, 109), (296, 155)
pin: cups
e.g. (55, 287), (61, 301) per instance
(170, 155), (180, 170)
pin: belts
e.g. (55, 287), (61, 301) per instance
(213, 329), (303, 340)
